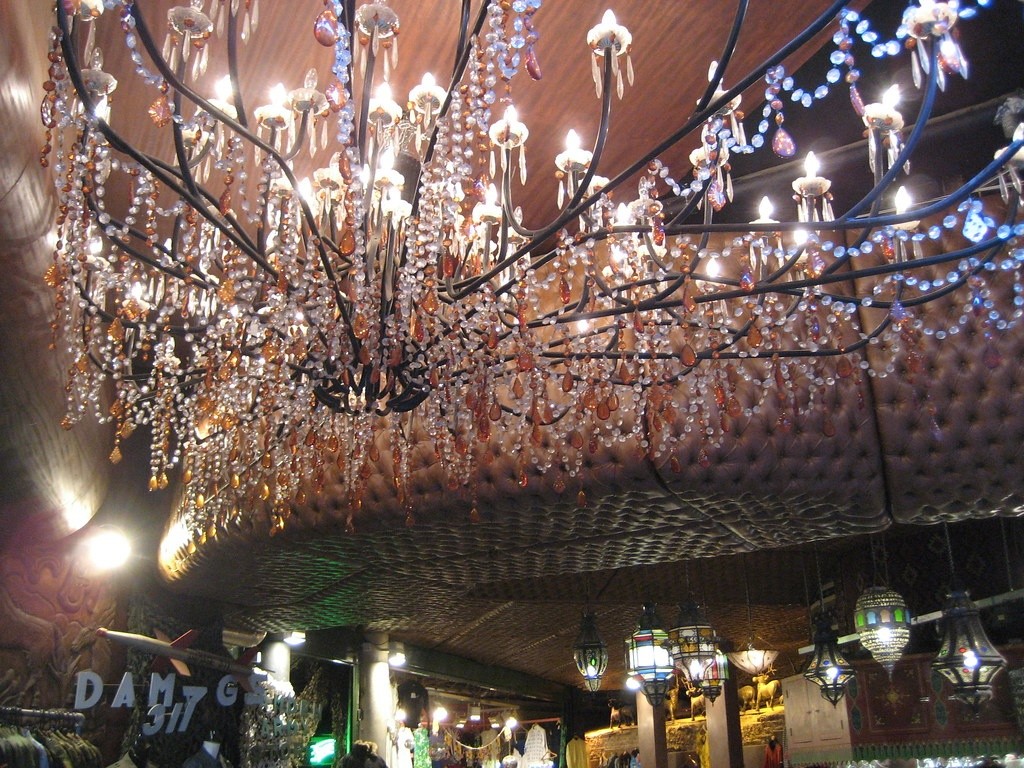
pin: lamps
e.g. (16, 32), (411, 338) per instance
(35, 0), (1023, 536)
(569, 523), (1007, 728)
